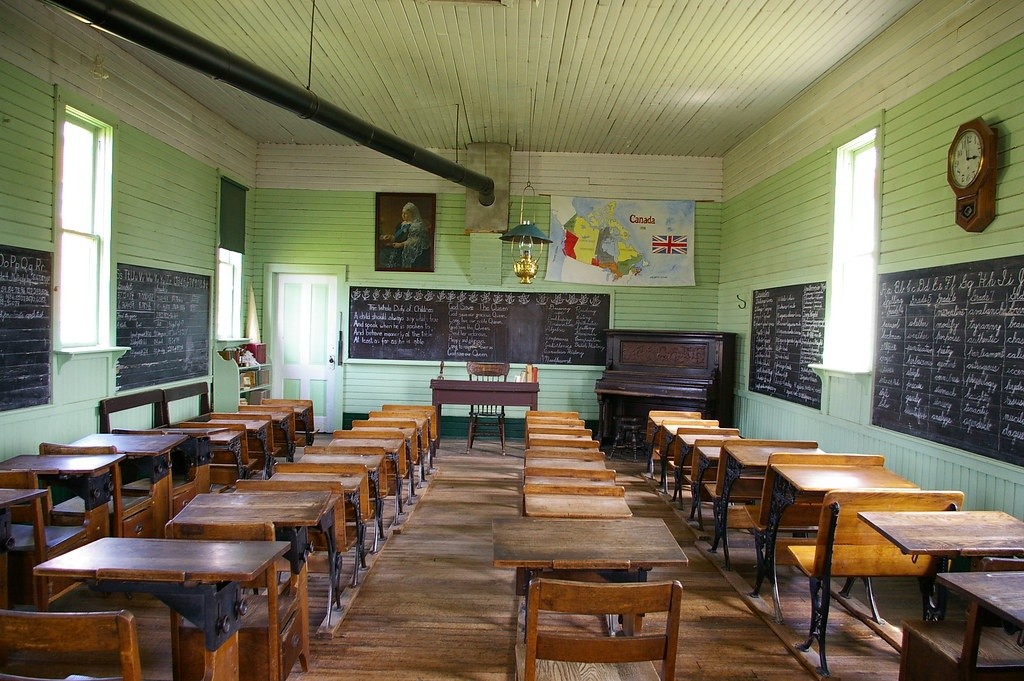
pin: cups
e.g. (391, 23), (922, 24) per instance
(532, 367), (537, 382)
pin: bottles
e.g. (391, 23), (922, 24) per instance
(527, 363), (532, 382)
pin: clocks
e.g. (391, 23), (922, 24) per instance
(947, 116), (998, 232)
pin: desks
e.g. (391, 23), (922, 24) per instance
(0, 379), (1024, 681)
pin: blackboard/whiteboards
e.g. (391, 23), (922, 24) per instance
(0, 245), (50, 411)
(871, 254), (1024, 468)
(344, 282), (615, 372)
(748, 281), (827, 411)
(115, 262), (211, 393)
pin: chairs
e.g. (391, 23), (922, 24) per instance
(687, 438), (721, 532)
(235, 479), (358, 587)
(705, 439), (819, 572)
(0, 468), (84, 611)
(514, 577), (683, 680)
(164, 520), (304, 680)
(274, 462), (377, 556)
(0, 609), (141, 681)
(110, 429), (195, 518)
(467, 361), (506, 456)
(39, 443), (151, 600)
(786, 490), (964, 678)
(667, 427), (739, 511)
(898, 558), (1024, 680)
(742, 452), (884, 624)
(655, 419), (720, 495)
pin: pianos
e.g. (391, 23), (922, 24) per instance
(594, 327), (739, 449)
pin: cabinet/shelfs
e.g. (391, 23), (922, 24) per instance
(214, 348), (272, 413)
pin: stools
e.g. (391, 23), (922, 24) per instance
(608, 421), (645, 463)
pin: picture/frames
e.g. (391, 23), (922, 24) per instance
(375, 192), (437, 272)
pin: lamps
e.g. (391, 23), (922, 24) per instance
(499, 90), (553, 283)
